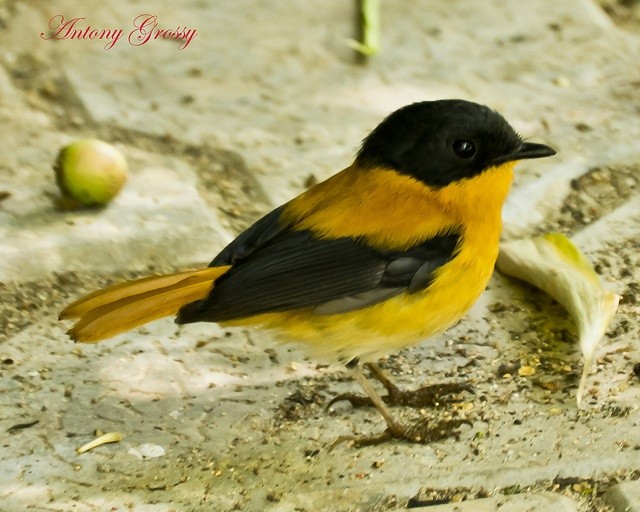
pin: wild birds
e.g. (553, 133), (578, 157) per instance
(59, 100), (556, 447)
